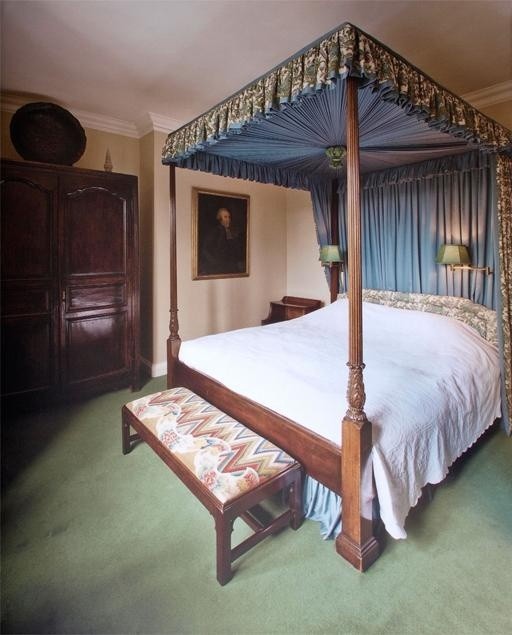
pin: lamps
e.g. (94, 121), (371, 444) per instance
(433, 244), (490, 277)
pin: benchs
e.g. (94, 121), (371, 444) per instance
(120, 386), (305, 586)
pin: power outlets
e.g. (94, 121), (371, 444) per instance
(320, 244), (343, 269)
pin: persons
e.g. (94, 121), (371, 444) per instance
(203, 205), (243, 275)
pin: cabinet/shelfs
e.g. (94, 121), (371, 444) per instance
(0, 157), (142, 417)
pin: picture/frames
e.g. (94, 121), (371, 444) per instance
(191, 182), (250, 279)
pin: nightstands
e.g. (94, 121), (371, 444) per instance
(261, 295), (321, 325)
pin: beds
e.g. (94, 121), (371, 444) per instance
(162, 23), (512, 572)
